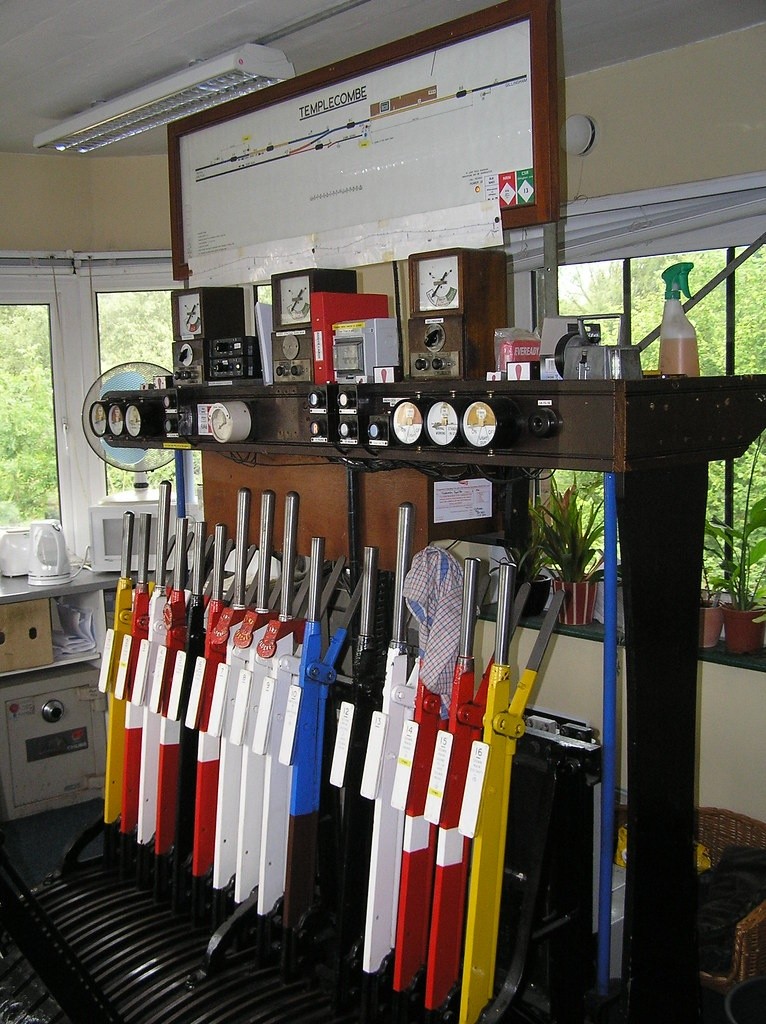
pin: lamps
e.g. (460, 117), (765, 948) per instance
(559, 113), (595, 157)
(33, 41), (295, 154)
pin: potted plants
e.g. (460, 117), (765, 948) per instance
(509, 481), (607, 625)
(695, 432), (766, 655)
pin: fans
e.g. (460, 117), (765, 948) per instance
(82, 361), (172, 503)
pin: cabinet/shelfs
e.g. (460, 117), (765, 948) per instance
(1, 565), (172, 822)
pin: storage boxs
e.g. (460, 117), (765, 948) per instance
(0, 598), (54, 673)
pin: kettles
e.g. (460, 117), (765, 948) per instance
(28, 520), (73, 585)
